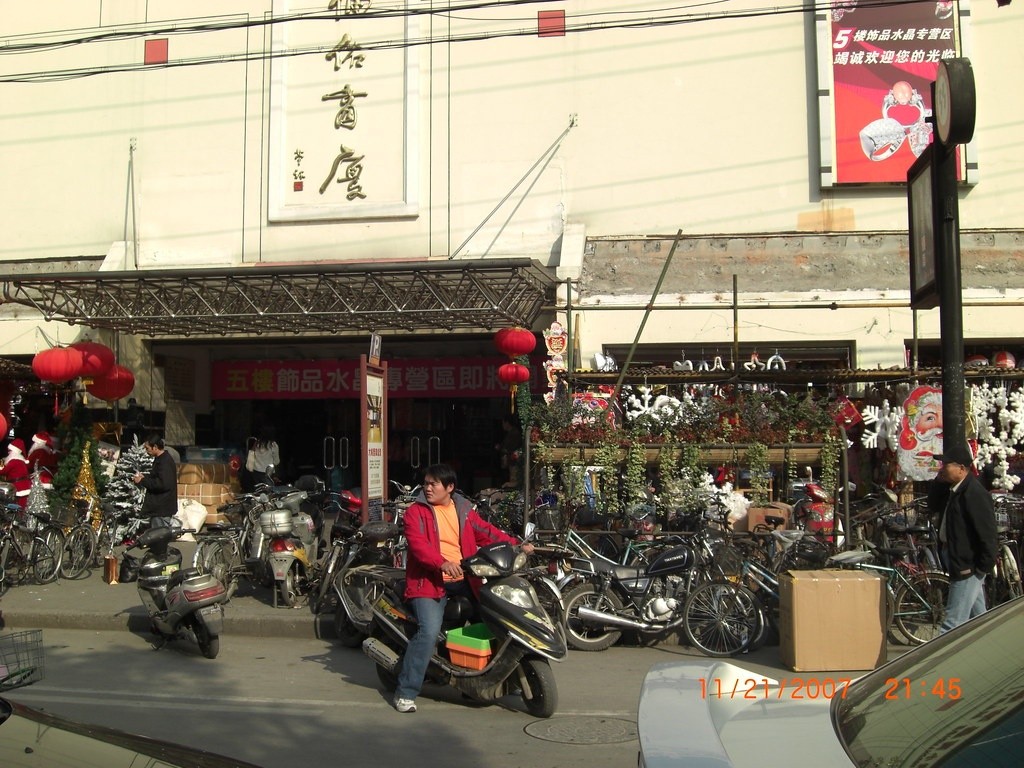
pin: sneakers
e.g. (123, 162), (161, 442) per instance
(393, 693), (416, 712)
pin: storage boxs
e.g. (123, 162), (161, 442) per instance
(775, 565), (888, 673)
(172, 457), (247, 524)
(445, 621), (498, 671)
(747, 498), (795, 534)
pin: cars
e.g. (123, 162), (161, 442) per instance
(636, 594), (1024, 766)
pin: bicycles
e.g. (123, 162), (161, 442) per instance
(195, 464), (1024, 656)
(0, 483), (118, 588)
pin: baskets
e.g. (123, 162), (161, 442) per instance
(225, 504), (272, 525)
(51, 506), (80, 526)
(0, 629), (45, 687)
(712, 544), (743, 576)
(1007, 504), (1024, 530)
(498, 500), (709, 533)
(794, 539), (832, 570)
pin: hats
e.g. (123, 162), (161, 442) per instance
(8, 438), (29, 464)
(32, 432), (54, 447)
(933, 446), (972, 468)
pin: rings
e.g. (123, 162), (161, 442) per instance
(935, 1), (953, 19)
(859, 119), (905, 163)
(882, 81), (926, 130)
(830, 0), (858, 22)
(909, 122), (934, 159)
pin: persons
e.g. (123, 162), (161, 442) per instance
(27, 431), (59, 491)
(394, 462), (535, 712)
(933, 447), (998, 634)
(495, 415), (521, 479)
(0, 439), (31, 513)
(245, 423), (280, 492)
(134, 435), (178, 527)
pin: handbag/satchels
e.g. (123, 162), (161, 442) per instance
(246, 449), (256, 472)
(104, 548), (119, 585)
(118, 551), (141, 583)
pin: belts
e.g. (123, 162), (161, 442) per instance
(9, 476), (29, 483)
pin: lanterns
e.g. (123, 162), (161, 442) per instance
(0, 408), (11, 442)
(32, 345), (83, 416)
(85, 365), (135, 409)
(69, 339), (116, 404)
(498, 361), (531, 414)
(496, 324), (536, 358)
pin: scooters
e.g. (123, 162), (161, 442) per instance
(125, 526), (227, 658)
(354, 540), (569, 717)
